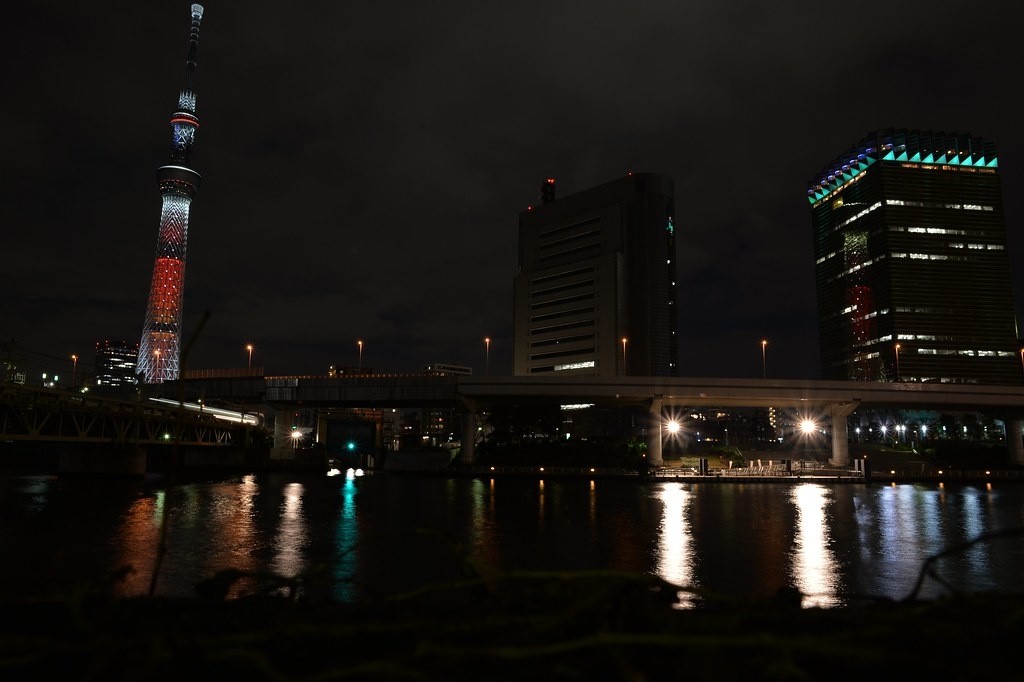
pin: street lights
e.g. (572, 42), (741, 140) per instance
(896, 345), (900, 382)
(72, 355), (77, 386)
(623, 338), (627, 375)
(248, 346), (252, 375)
(358, 341), (362, 376)
(155, 350), (159, 384)
(485, 338), (490, 376)
(762, 340), (767, 379)
